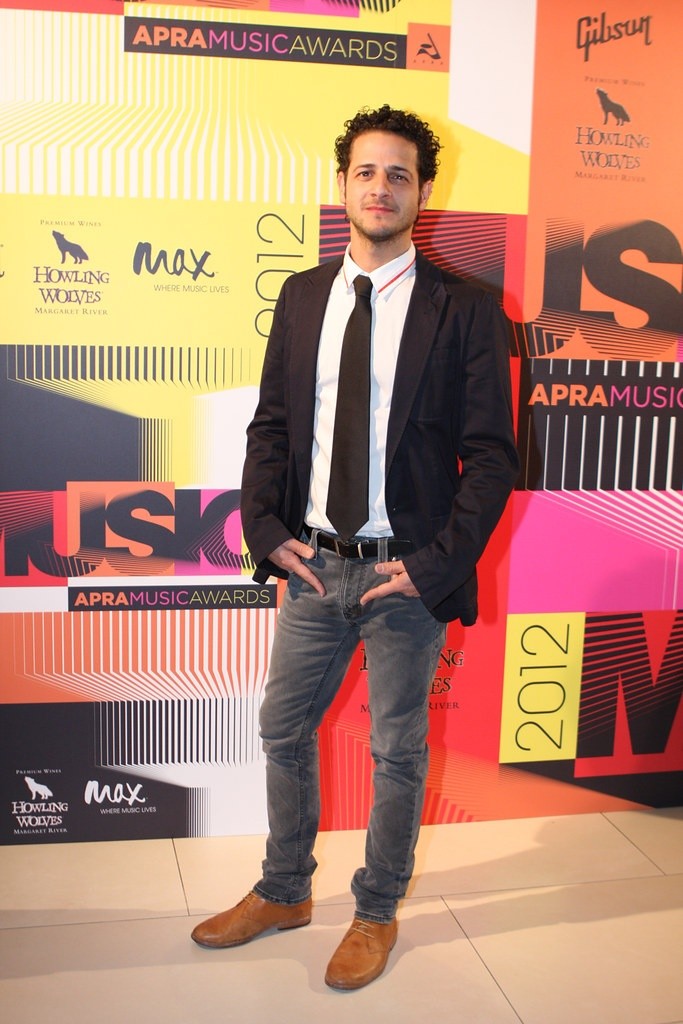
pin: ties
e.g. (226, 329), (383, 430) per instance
(325, 274), (373, 543)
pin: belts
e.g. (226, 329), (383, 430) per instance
(302, 522), (413, 558)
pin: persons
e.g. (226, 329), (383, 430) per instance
(190, 106), (523, 990)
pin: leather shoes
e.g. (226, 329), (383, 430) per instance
(190, 887), (312, 949)
(324, 913), (397, 989)
